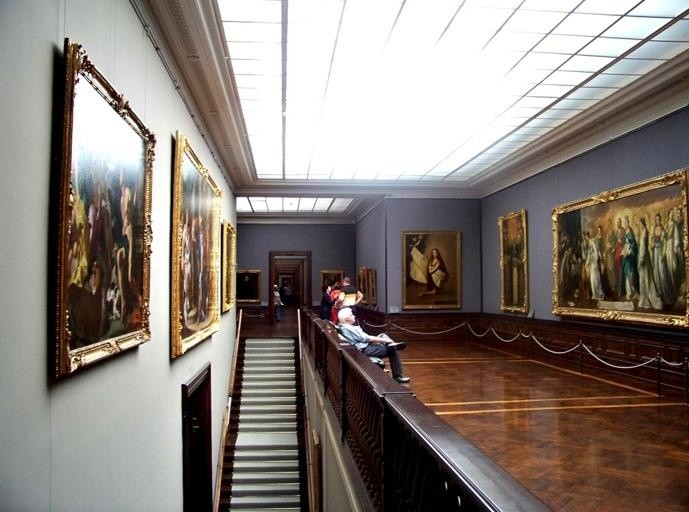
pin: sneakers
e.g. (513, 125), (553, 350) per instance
(388, 342), (406, 351)
(393, 376), (411, 383)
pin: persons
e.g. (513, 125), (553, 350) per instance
(320, 277), (410, 383)
(560, 204), (685, 310)
(273, 280), (289, 322)
(427, 248), (449, 290)
(506, 233), (524, 305)
(406, 235), (429, 284)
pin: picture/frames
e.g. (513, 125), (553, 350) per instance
(222, 219), (235, 312)
(170, 129), (222, 360)
(498, 208), (529, 314)
(51, 35), (157, 378)
(320, 270), (343, 291)
(551, 167), (689, 329)
(236, 268), (262, 303)
(358, 269), (376, 305)
(402, 231), (462, 310)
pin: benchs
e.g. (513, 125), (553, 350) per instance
(324, 316), (385, 372)
